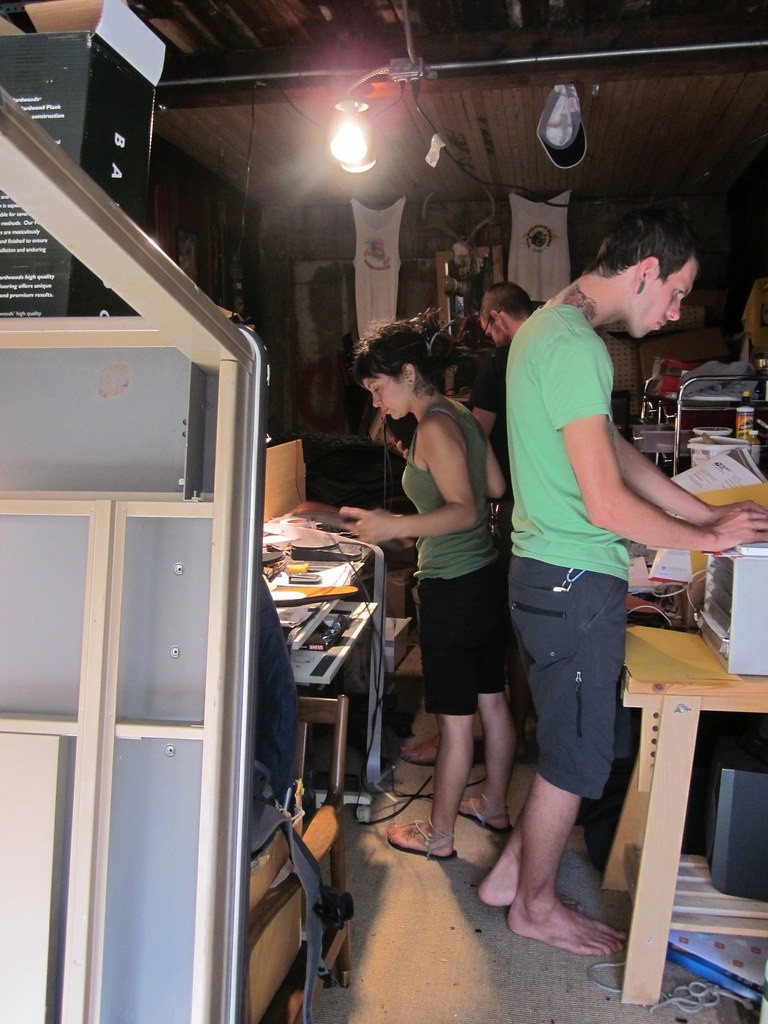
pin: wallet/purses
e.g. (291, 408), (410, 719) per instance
(627, 595), (665, 627)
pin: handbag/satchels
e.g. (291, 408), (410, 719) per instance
(646, 359), (700, 393)
(383, 412), (418, 511)
(488, 501), (513, 538)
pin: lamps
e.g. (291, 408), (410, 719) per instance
(327, 56), (425, 170)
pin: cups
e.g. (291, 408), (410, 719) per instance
(735, 407), (753, 440)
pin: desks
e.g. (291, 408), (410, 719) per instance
(600, 627), (767, 1005)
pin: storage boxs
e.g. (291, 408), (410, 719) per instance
(0, 0), (166, 316)
(384, 617), (413, 670)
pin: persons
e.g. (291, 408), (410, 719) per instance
(337, 280), (536, 863)
(474, 209), (768, 959)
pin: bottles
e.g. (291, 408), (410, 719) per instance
(747, 431), (760, 465)
(737, 390), (752, 406)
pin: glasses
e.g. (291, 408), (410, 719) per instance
(484, 309), (502, 336)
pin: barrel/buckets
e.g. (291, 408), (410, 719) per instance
(687, 436), (750, 472)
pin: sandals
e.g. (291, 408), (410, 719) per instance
(387, 816), (457, 860)
(458, 792), (513, 834)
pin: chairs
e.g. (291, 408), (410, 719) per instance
(237, 693), (352, 1024)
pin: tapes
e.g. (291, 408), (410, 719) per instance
(286, 560), (308, 574)
(280, 518), (308, 527)
